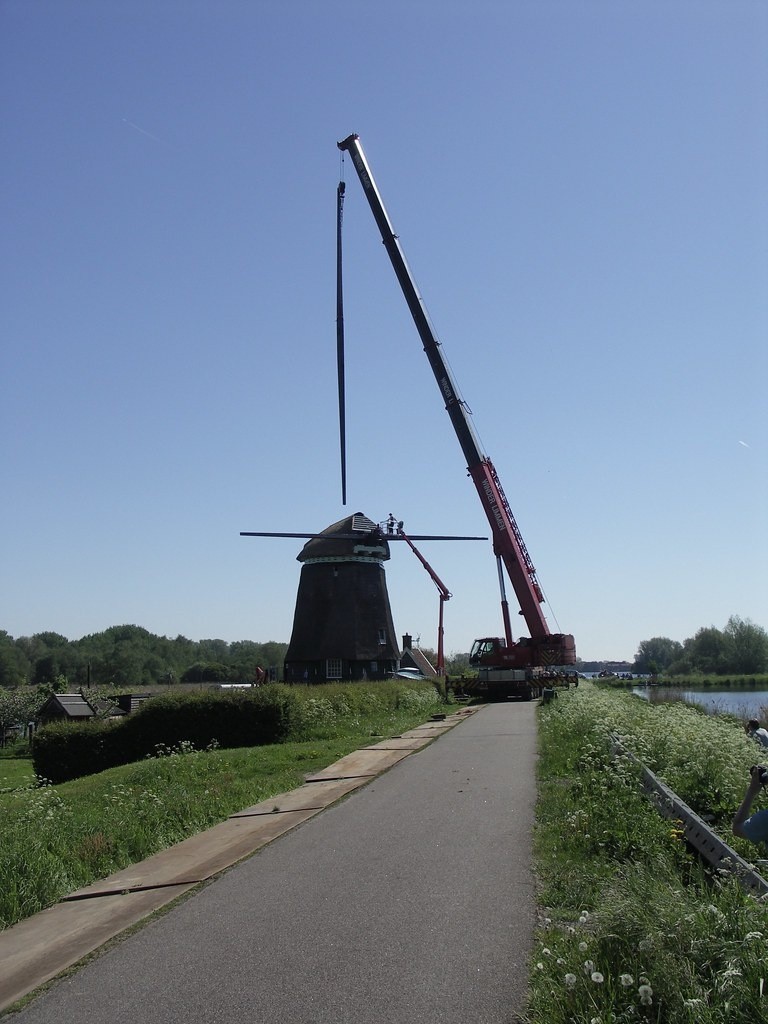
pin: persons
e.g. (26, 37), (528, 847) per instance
(731, 765), (768, 846)
(386, 513), (399, 535)
(744, 719), (768, 747)
(590, 670), (652, 680)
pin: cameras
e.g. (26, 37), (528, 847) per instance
(751, 765), (768, 784)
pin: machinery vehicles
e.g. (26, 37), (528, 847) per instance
(336, 131), (580, 705)
